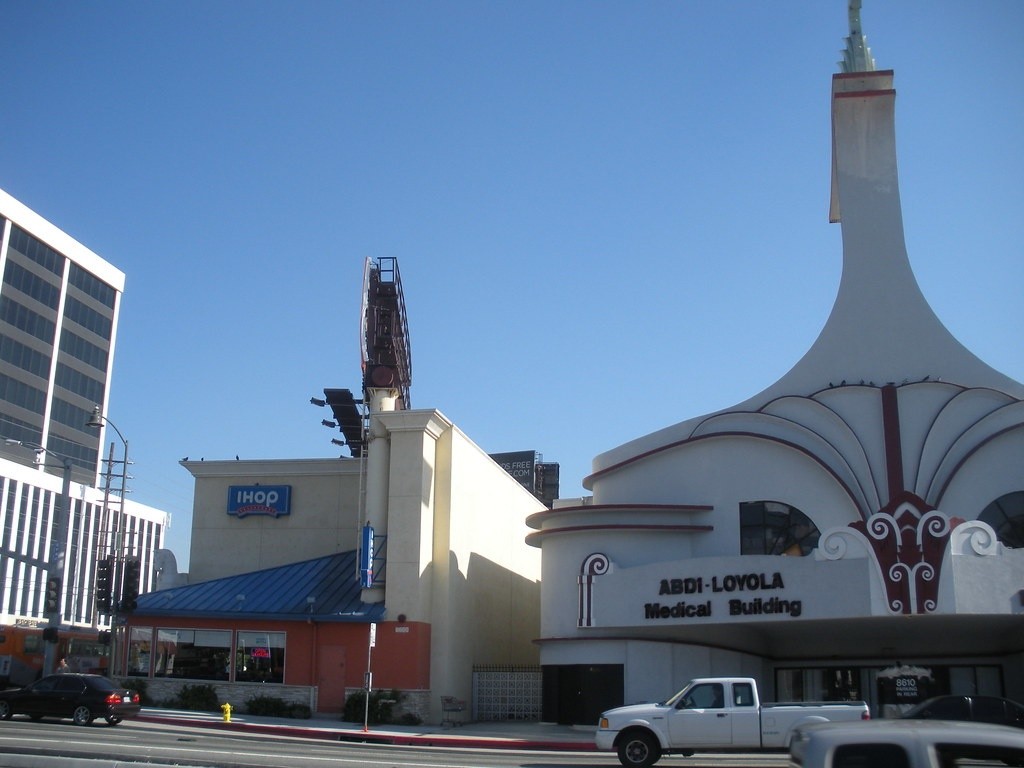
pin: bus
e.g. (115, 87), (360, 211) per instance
(0, 623), (111, 686)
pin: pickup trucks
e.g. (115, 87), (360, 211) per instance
(595, 678), (872, 768)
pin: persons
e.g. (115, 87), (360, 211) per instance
(57, 658), (70, 673)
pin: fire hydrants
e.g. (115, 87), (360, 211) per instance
(219, 703), (233, 722)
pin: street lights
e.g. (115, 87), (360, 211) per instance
(83, 404), (128, 678)
(5, 438), (73, 680)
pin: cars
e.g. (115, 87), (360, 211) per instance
(0, 674), (141, 726)
(787, 694), (1024, 768)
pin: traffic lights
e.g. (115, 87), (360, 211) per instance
(43, 628), (59, 643)
(44, 577), (59, 613)
(95, 555), (112, 612)
(98, 629), (109, 645)
(121, 556), (141, 611)
